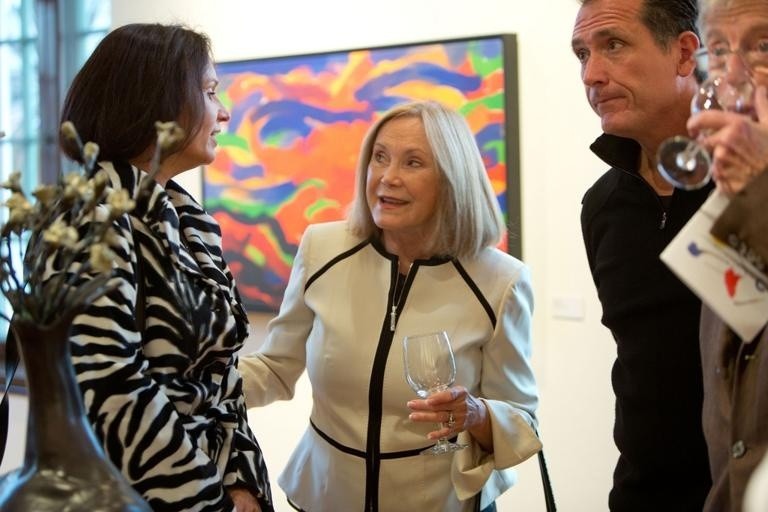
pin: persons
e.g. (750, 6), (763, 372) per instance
(679, 0), (766, 512)
(20, 21), (278, 512)
(569, 0), (723, 512)
(237, 95), (542, 512)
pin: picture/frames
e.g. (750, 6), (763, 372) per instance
(202, 34), (521, 312)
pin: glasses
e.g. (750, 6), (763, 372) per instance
(695, 47), (768, 75)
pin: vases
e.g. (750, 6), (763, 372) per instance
(0, 315), (153, 512)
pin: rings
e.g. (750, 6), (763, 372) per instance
(447, 412), (454, 428)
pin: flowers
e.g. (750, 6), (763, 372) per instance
(1, 120), (187, 325)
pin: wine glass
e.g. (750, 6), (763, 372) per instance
(655, 71), (760, 190)
(403, 330), (472, 455)
